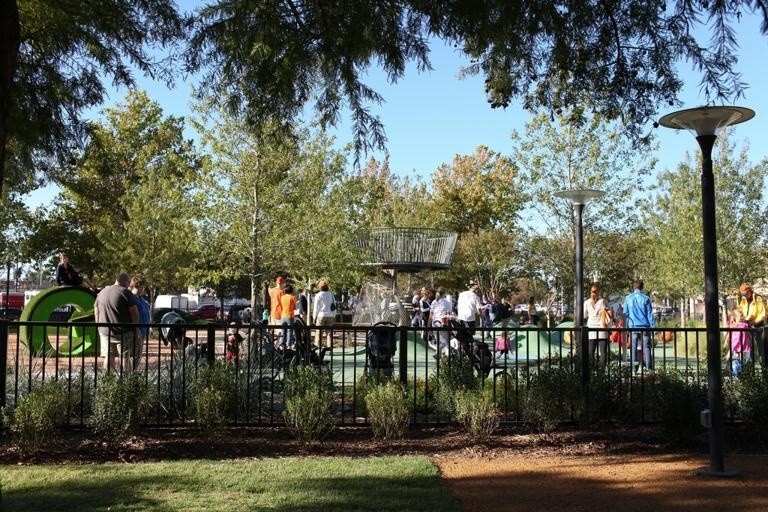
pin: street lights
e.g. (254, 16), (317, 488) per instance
(552, 183), (608, 360)
(657, 103), (758, 480)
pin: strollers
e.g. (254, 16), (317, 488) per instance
(362, 320), (397, 378)
(159, 311), (207, 357)
(245, 314), (331, 370)
(443, 318), (512, 387)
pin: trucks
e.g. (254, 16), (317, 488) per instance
(0, 291), (25, 319)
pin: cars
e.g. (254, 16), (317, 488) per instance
(153, 291), (251, 322)
(651, 302), (680, 320)
(401, 296), (414, 313)
(513, 299), (566, 318)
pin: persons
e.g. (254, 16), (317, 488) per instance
(55, 252), (101, 293)
(584, 280), (654, 372)
(129, 276), (152, 361)
(722, 284), (768, 376)
(267, 276), (336, 348)
(405, 286), (513, 357)
(528, 300), (537, 324)
(349, 290), (396, 312)
(223, 309), (256, 364)
(93, 273), (140, 374)
